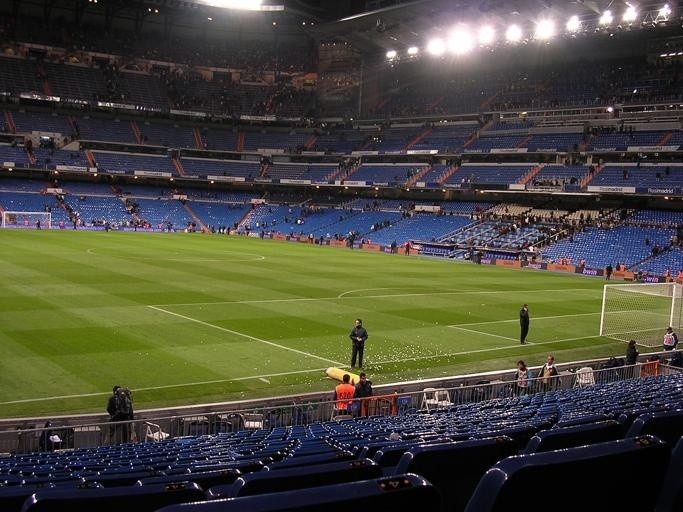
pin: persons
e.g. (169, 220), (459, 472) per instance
(519, 303), (529, 344)
(351, 372), (374, 418)
(39, 420), (74, 451)
(332, 374), (355, 420)
(349, 317), (368, 368)
(624, 339), (638, 378)
(516, 359), (533, 396)
(106, 385), (134, 444)
(661, 325), (679, 351)
(535, 355), (562, 391)
(1, 15), (683, 285)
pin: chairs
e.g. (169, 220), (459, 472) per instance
(1, 13), (683, 277)
(0, 351), (683, 512)
